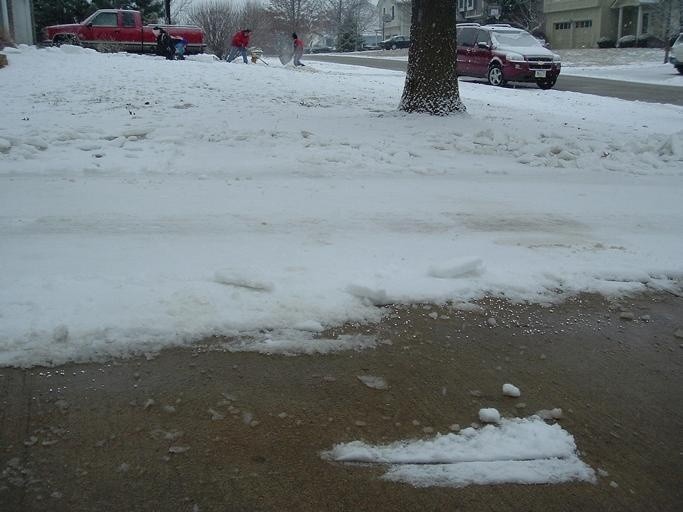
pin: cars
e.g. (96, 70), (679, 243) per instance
(377, 35), (411, 51)
(246, 46), (266, 57)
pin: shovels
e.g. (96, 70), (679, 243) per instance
(278, 52), (294, 65)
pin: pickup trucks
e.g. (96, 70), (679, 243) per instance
(38, 9), (208, 56)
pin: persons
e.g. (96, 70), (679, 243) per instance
(292, 32), (306, 66)
(152, 26), (187, 61)
(227, 28), (252, 65)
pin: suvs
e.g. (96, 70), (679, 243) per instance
(455, 22), (561, 92)
